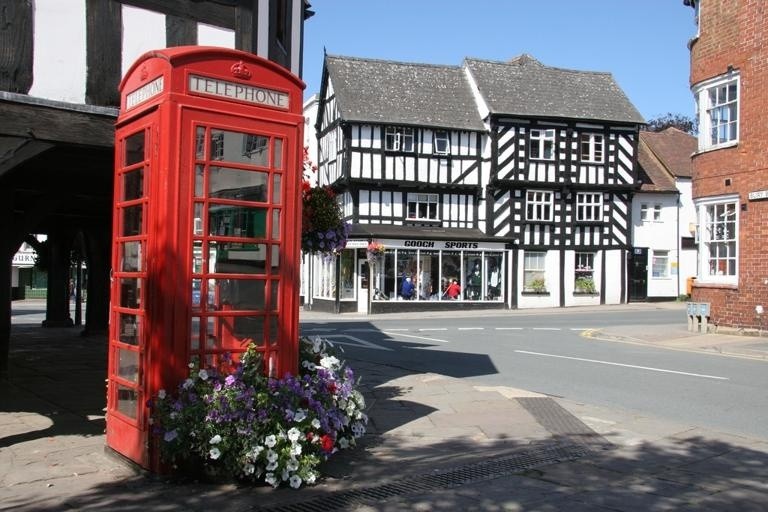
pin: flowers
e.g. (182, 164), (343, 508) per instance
(364, 239), (386, 257)
(300, 145), (351, 262)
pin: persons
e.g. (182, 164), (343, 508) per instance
(488, 263), (500, 299)
(446, 280), (460, 297)
(399, 274), (414, 300)
(470, 262), (480, 298)
